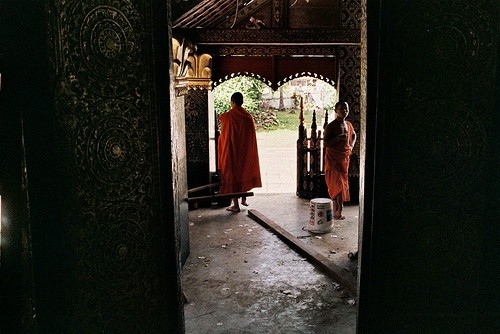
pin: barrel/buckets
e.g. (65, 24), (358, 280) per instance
(308, 198), (335, 233)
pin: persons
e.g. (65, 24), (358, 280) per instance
(219, 92), (263, 213)
(325, 101), (356, 219)
